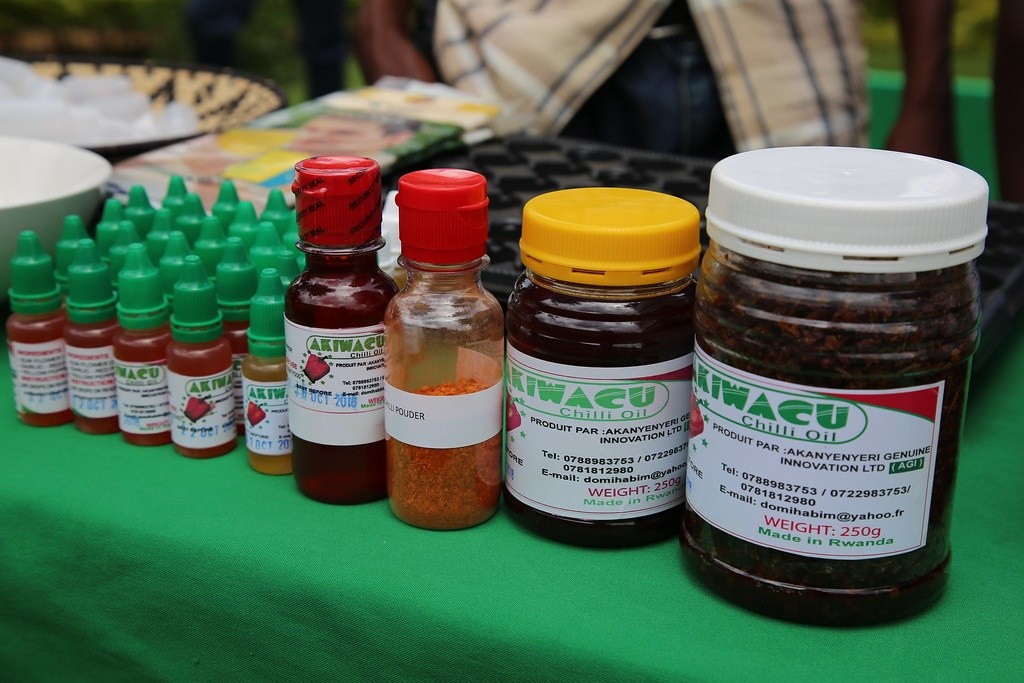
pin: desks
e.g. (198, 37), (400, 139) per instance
(0, 348), (1023, 683)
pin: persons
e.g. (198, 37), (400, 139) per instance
(341, 0), (958, 173)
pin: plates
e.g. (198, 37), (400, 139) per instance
(31, 61), (280, 162)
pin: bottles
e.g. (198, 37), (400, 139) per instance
(383, 168), (504, 533)
(284, 154), (400, 506)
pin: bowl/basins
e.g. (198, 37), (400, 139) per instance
(0, 132), (112, 296)
